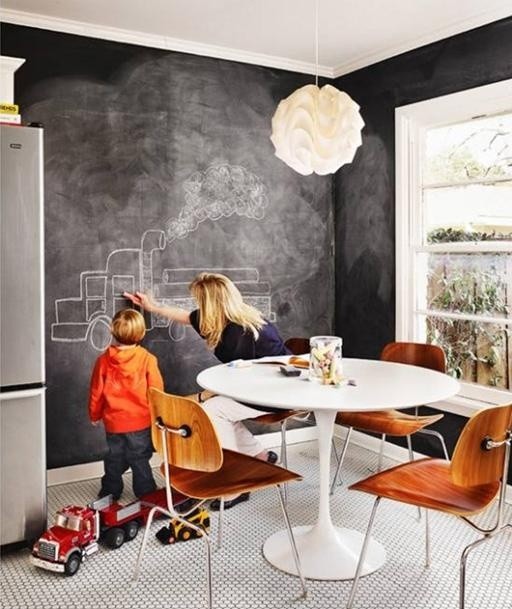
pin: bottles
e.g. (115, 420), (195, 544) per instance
(309, 335), (343, 386)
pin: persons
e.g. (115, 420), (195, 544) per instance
(123, 272), (301, 510)
(89, 307), (164, 502)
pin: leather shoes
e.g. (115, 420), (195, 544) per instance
(210, 493), (249, 511)
(267, 452), (277, 463)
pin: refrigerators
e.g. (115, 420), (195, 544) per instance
(1, 121), (46, 556)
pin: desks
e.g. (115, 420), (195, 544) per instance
(196, 349), (465, 585)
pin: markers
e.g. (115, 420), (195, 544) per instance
(122, 291), (140, 302)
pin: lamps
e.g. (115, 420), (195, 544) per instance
(265, 1), (370, 175)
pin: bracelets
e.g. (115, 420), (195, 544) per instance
(199, 392), (204, 403)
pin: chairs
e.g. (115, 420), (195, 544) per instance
(127, 383), (312, 608)
(346, 401), (512, 609)
(238, 336), (344, 508)
(328, 339), (452, 521)
